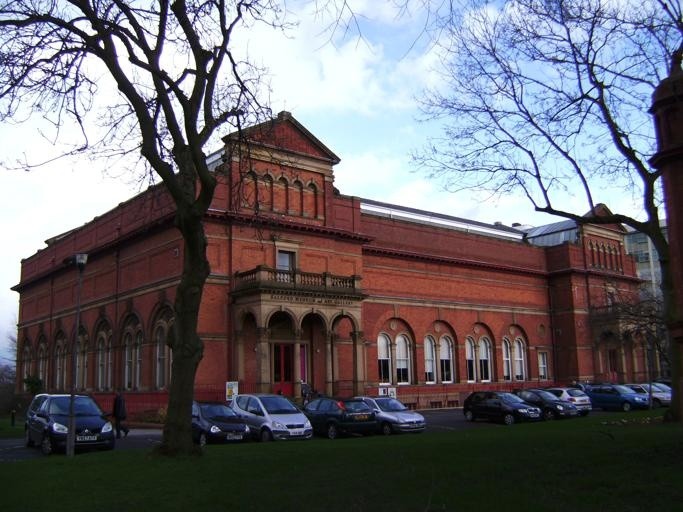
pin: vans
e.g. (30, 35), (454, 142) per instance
(461, 389), (541, 426)
(23, 390), (115, 456)
(228, 392), (314, 445)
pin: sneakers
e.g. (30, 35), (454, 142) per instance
(116, 435), (121, 439)
(124, 430), (129, 438)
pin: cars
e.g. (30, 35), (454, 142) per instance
(652, 379), (673, 396)
(579, 384), (652, 414)
(183, 399), (251, 449)
(628, 383), (671, 408)
(353, 395), (427, 438)
(512, 387), (578, 422)
(296, 396), (376, 441)
(545, 384), (592, 420)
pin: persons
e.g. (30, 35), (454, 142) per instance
(111, 386), (130, 440)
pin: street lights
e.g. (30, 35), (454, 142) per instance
(67, 250), (89, 457)
